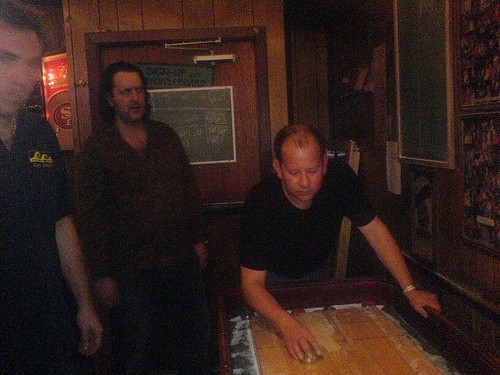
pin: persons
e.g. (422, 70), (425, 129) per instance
(237, 123), (441, 362)
(0, 0), (104, 374)
(73, 61), (220, 375)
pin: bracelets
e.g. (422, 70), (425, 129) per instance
(403, 283), (417, 295)
(201, 239), (209, 246)
